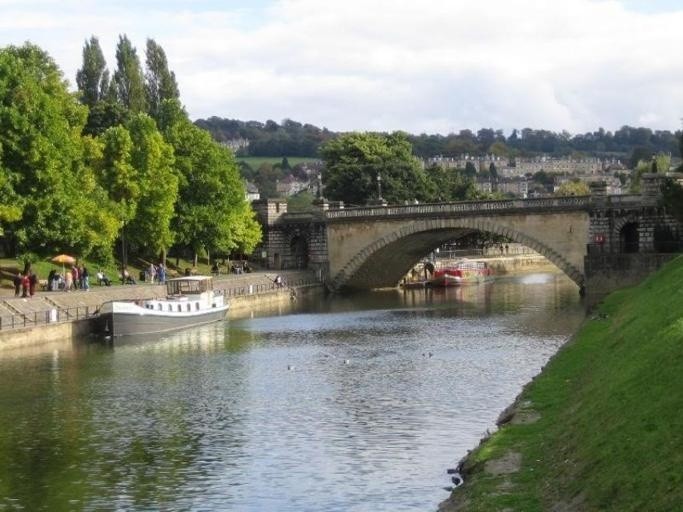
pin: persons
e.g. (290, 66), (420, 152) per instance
(41, 264), (90, 291)
(146, 264), (156, 284)
(20, 273), (29, 297)
(96, 269), (112, 286)
(27, 270), (37, 296)
(13, 272), (22, 297)
(124, 266), (136, 283)
(156, 263), (165, 286)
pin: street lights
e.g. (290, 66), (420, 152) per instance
(314, 173), (322, 196)
(375, 173), (382, 200)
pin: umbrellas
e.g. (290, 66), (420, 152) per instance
(49, 253), (75, 275)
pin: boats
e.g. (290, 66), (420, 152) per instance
(429, 257), (496, 286)
(95, 273), (230, 338)
(399, 262), (434, 287)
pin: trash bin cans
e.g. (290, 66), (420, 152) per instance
(248, 284), (254, 294)
(48, 269), (59, 290)
(184, 267), (190, 276)
(48, 308), (57, 321)
(139, 272), (145, 281)
